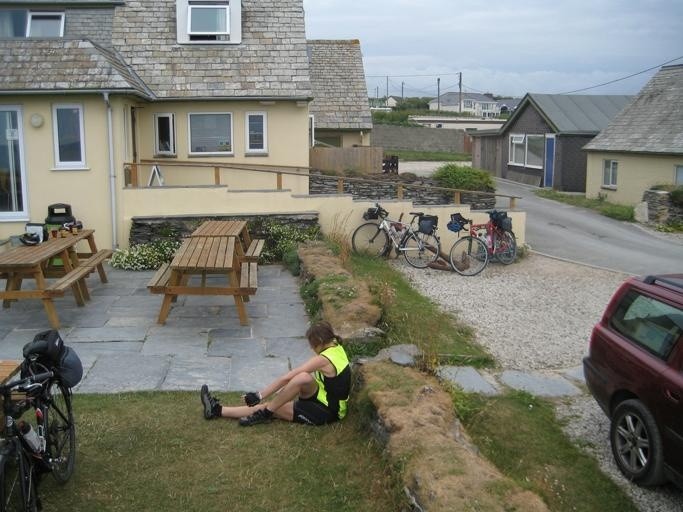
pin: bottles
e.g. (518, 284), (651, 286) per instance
(17, 421), (41, 454)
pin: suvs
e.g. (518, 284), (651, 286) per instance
(581, 270), (681, 492)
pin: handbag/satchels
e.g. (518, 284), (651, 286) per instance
(21, 330), (82, 387)
(419, 215), (437, 233)
(487, 212), (511, 234)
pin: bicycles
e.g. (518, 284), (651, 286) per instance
(0, 329), (78, 512)
(447, 207), (519, 275)
(350, 199), (440, 270)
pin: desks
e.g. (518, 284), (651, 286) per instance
(190, 220), (253, 265)
(1, 359), (24, 441)
(13, 229), (107, 301)
(156, 237), (249, 326)
(0, 237), (85, 329)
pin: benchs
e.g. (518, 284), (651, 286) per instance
(245, 238), (266, 262)
(238, 262), (258, 296)
(79, 249), (115, 271)
(146, 261), (175, 295)
(1, 390), (29, 402)
(44, 267), (92, 299)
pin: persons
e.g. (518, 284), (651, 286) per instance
(200, 320), (352, 427)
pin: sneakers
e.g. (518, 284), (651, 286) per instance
(201, 386), (219, 419)
(241, 409), (272, 426)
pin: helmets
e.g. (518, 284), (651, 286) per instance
(448, 223), (461, 231)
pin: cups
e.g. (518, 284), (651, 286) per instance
(51, 230), (58, 239)
(71, 226), (77, 235)
(59, 229), (67, 239)
